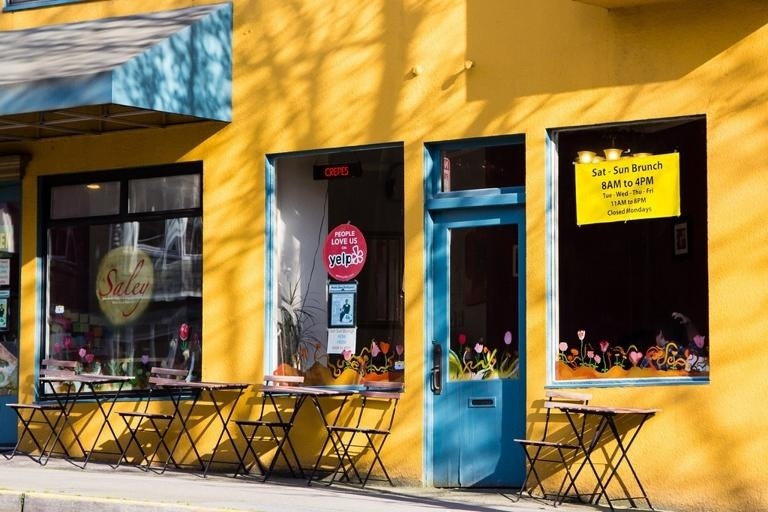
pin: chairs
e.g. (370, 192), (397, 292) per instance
(4, 357), (88, 461)
(103, 366), (189, 472)
(324, 379), (403, 488)
(234, 375), (305, 478)
(508, 389), (594, 506)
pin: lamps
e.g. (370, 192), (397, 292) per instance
(571, 127), (654, 170)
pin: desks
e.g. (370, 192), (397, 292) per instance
(155, 381), (250, 478)
(539, 400), (664, 512)
(252, 385), (363, 487)
(36, 374), (138, 468)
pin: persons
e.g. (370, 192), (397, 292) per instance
(339, 299), (350, 322)
(640, 311), (708, 369)
